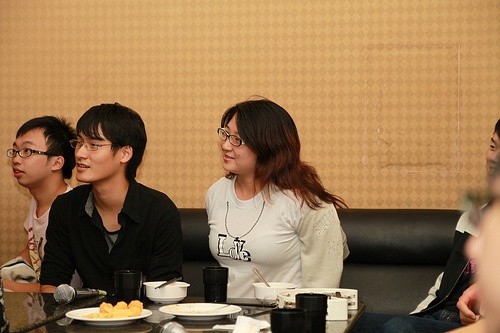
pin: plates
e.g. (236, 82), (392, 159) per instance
(159, 319), (235, 332)
(159, 302), (242, 321)
(66, 307), (152, 325)
(66, 325), (153, 333)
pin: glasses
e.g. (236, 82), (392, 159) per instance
(69, 138), (126, 151)
(6, 149), (60, 158)
(465, 188), (500, 226)
(217, 127), (246, 147)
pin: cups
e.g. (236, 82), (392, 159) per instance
(203, 266), (229, 302)
(276, 291), (297, 308)
(270, 308), (310, 333)
(113, 269), (141, 305)
(295, 292), (327, 333)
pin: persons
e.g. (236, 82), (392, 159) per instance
(7, 116), (85, 289)
(38, 103), (184, 293)
(204, 99), (344, 297)
(351, 120), (500, 333)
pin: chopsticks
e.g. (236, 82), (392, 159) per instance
(154, 275), (176, 289)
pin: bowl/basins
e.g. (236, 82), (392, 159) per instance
(144, 304), (175, 324)
(143, 281), (190, 304)
(252, 282), (297, 305)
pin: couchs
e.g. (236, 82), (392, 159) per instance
(176, 206), (465, 315)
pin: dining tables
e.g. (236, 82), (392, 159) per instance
(0, 270), (366, 333)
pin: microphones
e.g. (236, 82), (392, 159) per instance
(53, 284), (108, 305)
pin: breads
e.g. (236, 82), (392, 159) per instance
(83, 300), (143, 318)
